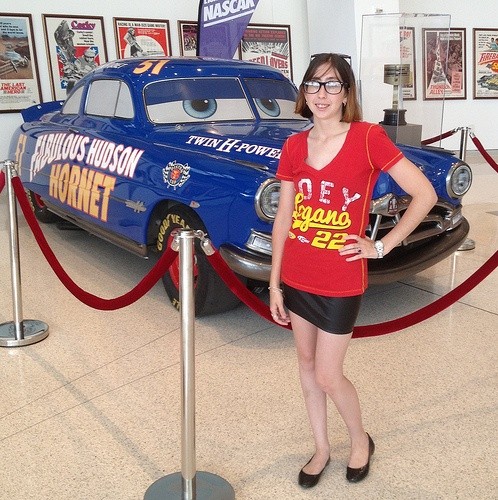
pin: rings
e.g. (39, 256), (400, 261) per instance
(357, 248), (361, 253)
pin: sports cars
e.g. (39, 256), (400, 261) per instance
(11, 54), (474, 316)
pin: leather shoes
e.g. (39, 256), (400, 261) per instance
(299, 453), (331, 488)
(346, 433), (375, 482)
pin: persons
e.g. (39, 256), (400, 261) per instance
(269, 54), (438, 489)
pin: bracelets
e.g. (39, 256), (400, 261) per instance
(268, 287), (282, 293)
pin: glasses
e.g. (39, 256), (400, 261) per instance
(302, 80), (346, 95)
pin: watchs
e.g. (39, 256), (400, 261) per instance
(375, 240), (385, 257)
